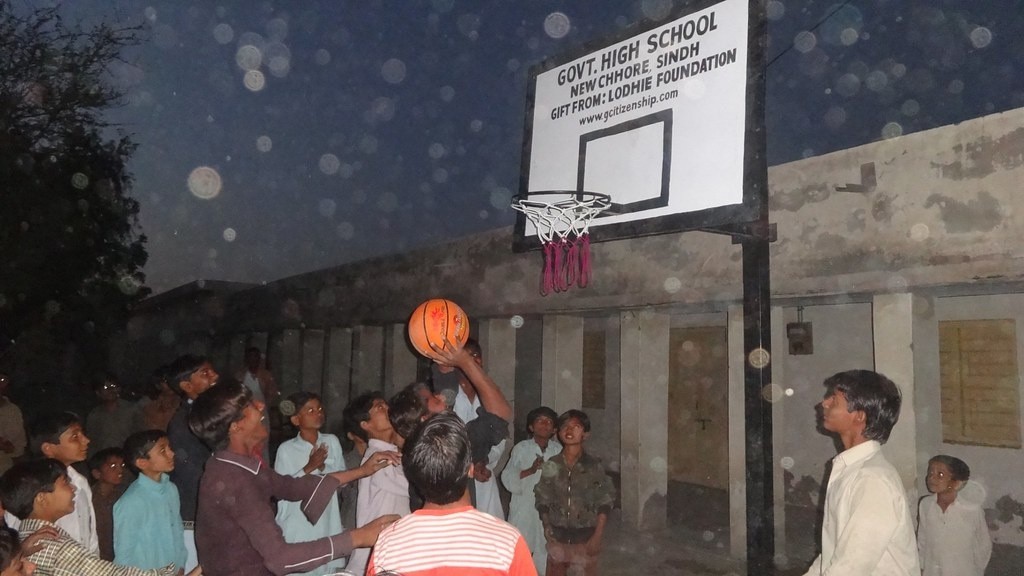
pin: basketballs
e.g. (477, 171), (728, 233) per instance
(408, 299), (470, 359)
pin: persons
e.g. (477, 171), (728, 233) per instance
(2, 329), (540, 576)
(914, 455), (992, 576)
(499, 406), (562, 576)
(802, 368), (923, 576)
(533, 408), (616, 576)
